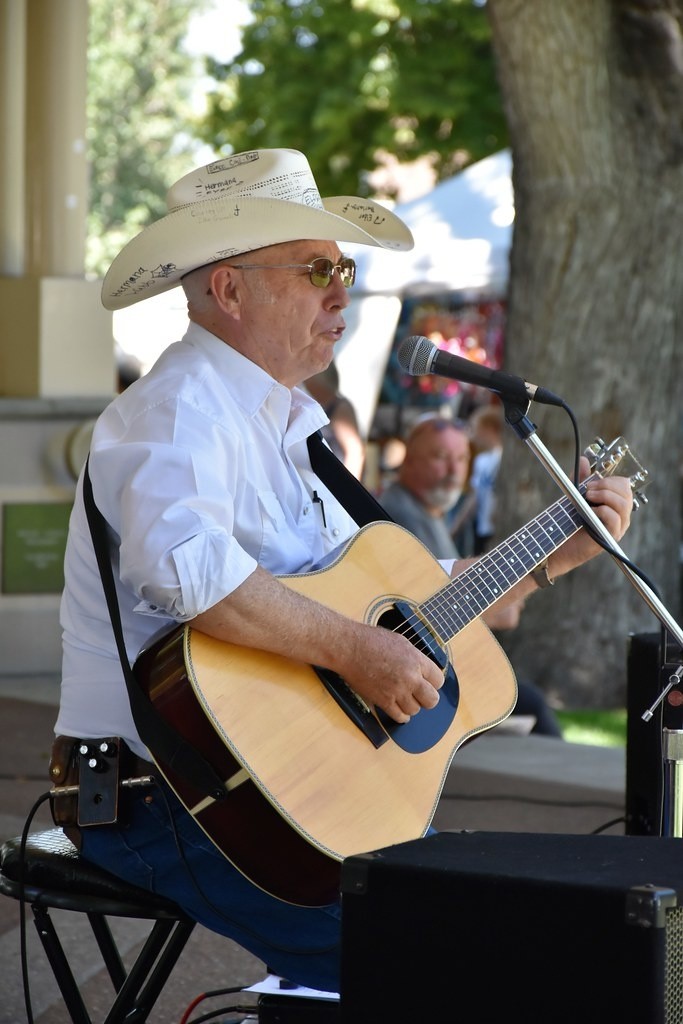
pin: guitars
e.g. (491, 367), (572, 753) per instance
(131, 436), (652, 904)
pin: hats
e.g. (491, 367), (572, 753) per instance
(100, 148), (414, 310)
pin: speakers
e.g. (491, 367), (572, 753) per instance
(335, 832), (683, 1024)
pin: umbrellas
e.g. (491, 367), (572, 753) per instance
(338, 148), (517, 299)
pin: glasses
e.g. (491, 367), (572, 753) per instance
(206, 257), (356, 295)
(413, 417), (466, 438)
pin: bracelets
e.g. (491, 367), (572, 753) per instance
(530, 559), (554, 588)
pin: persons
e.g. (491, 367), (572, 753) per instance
(51, 148), (634, 993)
(304, 360), (504, 557)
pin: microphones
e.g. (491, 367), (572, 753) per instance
(395, 336), (564, 408)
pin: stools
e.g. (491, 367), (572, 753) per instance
(0, 828), (196, 1024)
